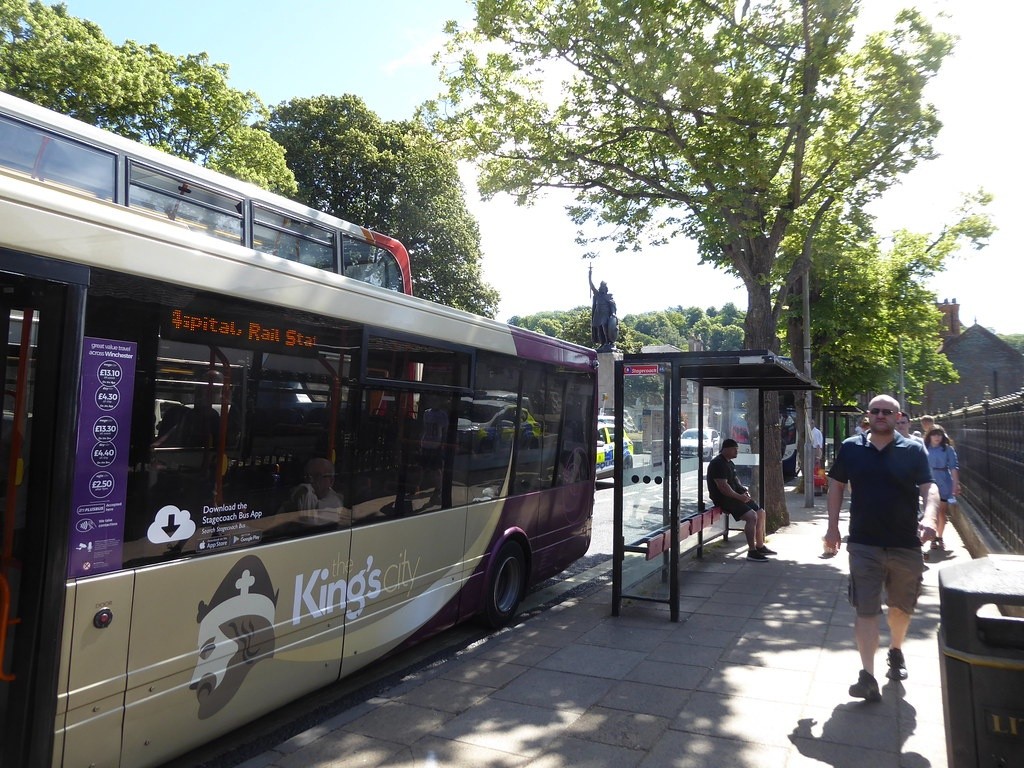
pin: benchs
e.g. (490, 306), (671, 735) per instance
(627, 507), (722, 561)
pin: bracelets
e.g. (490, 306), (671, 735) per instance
(954, 482), (960, 488)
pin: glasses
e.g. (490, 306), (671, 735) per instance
(870, 408), (897, 416)
(897, 421), (909, 424)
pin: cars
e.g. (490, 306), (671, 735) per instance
(445, 396), (541, 472)
(481, 389), (534, 418)
(679, 429), (723, 462)
(593, 415), (635, 478)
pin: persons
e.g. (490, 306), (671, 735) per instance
(680, 422), (685, 434)
(823, 395), (940, 702)
(289, 458), (340, 527)
(810, 419), (824, 496)
(588, 270), (616, 348)
(893, 412), (962, 560)
(707, 438), (776, 562)
(854, 417), (871, 434)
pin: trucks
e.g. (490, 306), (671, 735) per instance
(597, 407), (643, 453)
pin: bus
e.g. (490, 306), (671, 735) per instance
(0, 168), (600, 768)
(0, 91), (421, 420)
(726, 357), (804, 481)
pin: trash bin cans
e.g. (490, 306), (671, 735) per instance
(936, 554), (1024, 768)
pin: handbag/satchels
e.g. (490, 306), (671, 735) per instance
(813, 463), (825, 486)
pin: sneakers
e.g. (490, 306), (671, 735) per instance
(887, 648), (908, 679)
(756, 546), (777, 555)
(849, 670), (881, 701)
(748, 550), (769, 561)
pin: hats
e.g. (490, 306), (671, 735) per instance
(720, 439), (738, 452)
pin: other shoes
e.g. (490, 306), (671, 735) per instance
(930, 540), (937, 549)
(814, 492), (822, 496)
(939, 539), (944, 547)
